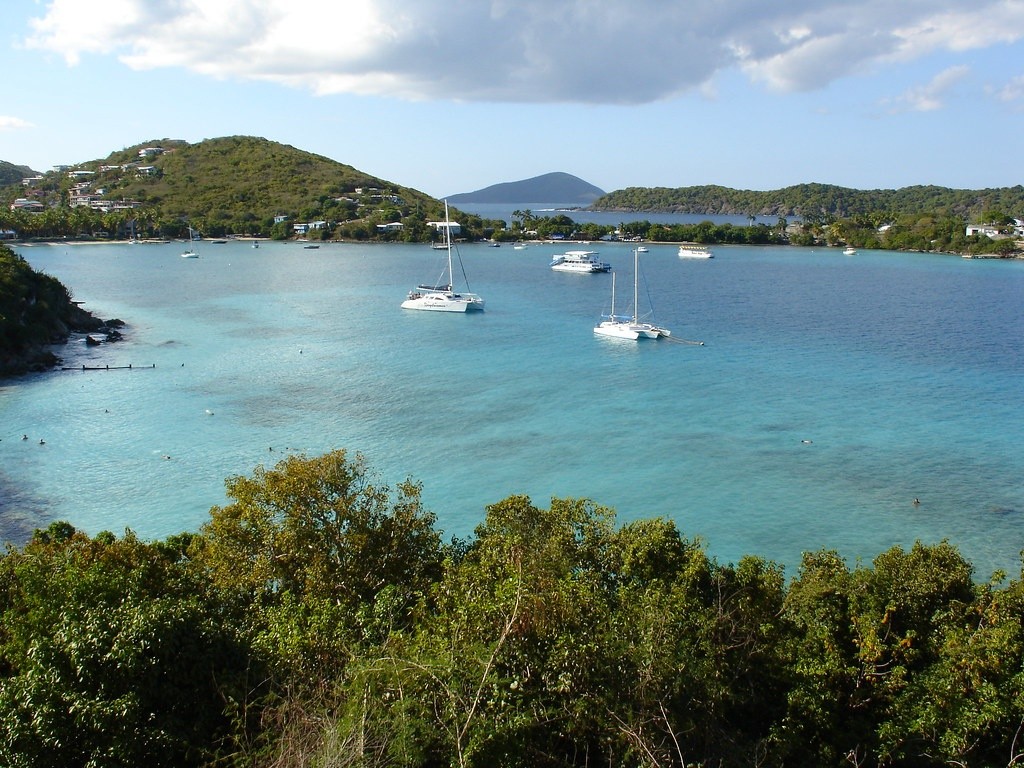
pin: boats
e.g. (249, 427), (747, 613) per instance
(513, 245), (528, 250)
(549, 250), (613, 273)
(304, 244), (320, 249)
(632, 246), (649, 252)
(677, 245), (716, 258)
(251, 241), (259, 248)
(487, 243), (501, 247)
(843, 247), (856, 255)
(211, 240), (228, 244)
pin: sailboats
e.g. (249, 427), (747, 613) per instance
(593, 248), (671, 341)
(179, 223), (201, 259)
(430, 221), (454, 250)
(399, 198), (485, 313)
